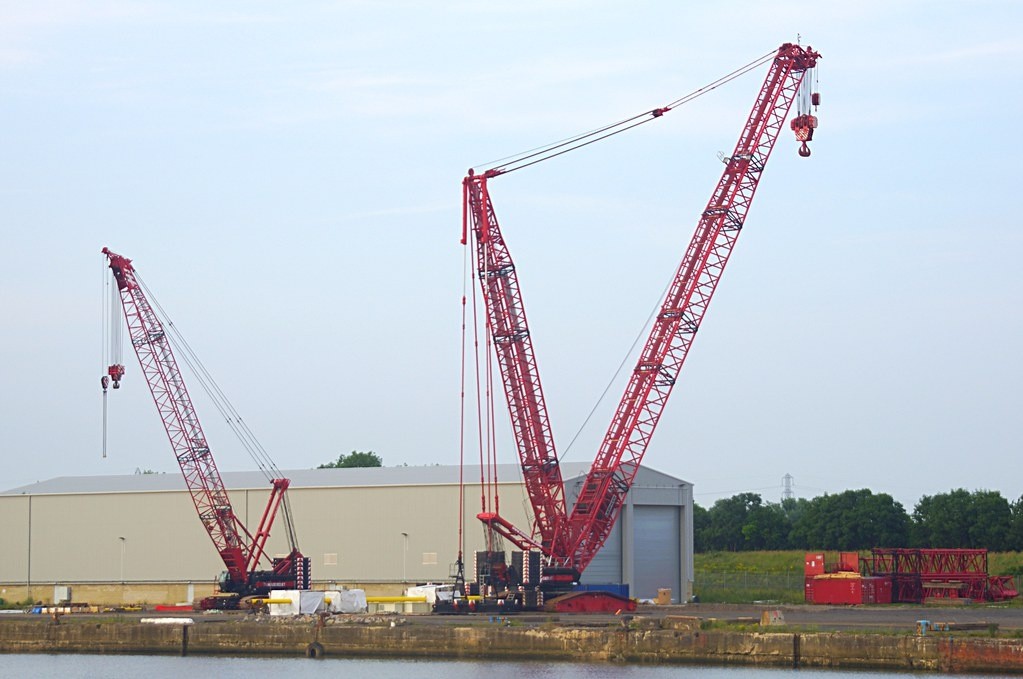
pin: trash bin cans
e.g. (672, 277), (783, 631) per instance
(657, 588), (671, 606)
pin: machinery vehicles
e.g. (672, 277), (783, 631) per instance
(99, 245), (311, 613)
(458, 43), (822, 616)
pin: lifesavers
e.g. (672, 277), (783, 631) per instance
(53, 612), (61, 622)
(305, 642), (323, 657)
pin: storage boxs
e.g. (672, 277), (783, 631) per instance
(803, 551), (892, 604)
(572, 584), (629, 599)
